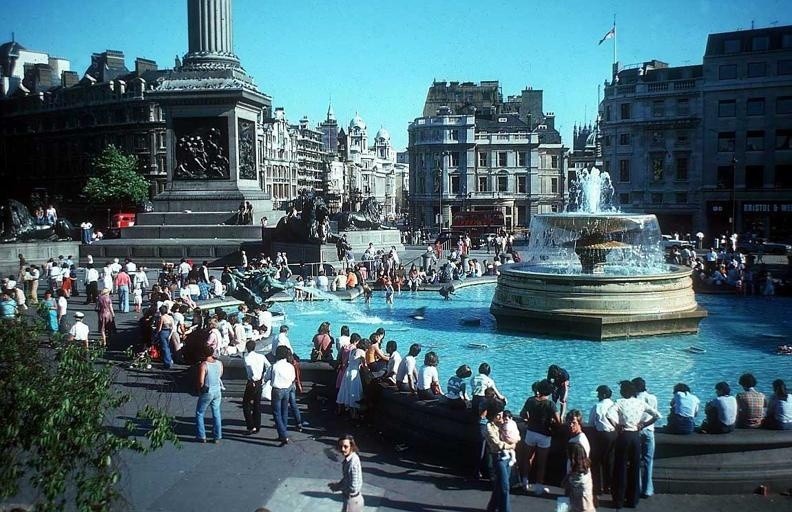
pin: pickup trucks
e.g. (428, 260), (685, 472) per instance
(735, 237), (791, 254)
(661, 234), (689, 250)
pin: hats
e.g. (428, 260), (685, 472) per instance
(101, 288), (109, 295)
(73, 312), (85, 319)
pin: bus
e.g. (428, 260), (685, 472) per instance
(112, 213), (135, 230)
(452, 209), (503, 236)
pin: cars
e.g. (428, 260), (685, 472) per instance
(482, 233), (498, 244)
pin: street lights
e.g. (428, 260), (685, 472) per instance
(728, 153), (739, 235)
(436, 168), (443, 235)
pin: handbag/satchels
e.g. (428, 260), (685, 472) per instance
(311, 347), (322, 361)
(296, 381), (302, 393)
(432, 382), (440, 396)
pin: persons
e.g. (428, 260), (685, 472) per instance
(0, 192), (791, 433)
(190, 345), (228, 447)
(327, 433), (366, 512)
(498, 410), (523, 468)
(604, 379), (665, 512)
(484, 404), (517, 512)
(270, 343), (297, 449)
(560, 440), (597, 512)
(630, 376), (663, 502)
(588, 383), (620, 498)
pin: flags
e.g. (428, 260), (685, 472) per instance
(598, 26), (616, 46)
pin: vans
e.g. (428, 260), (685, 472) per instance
(435, 232), (487, 250)
